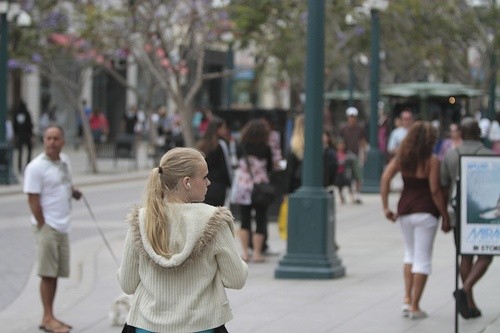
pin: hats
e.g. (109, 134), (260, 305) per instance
(346, 106), (358, 116)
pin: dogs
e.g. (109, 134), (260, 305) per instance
(104, 292), (136, 330)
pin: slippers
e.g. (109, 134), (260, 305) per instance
(39, 321), (72, 328)
(44, 323), (69, 333)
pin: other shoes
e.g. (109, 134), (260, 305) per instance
(469, 308), (480, 316)
(454, 289), (470, 318)
(402, 303), (411, 316)
(409, 310), (428, 318)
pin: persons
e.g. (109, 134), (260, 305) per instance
(234, 118), (274, 263)
(22, 125), (82, 332)
(119, 145), (249, 332)
(11, 99), (33, 169)
(40, 99), (500, 209)
(439, 117), (499, 318)
(380, 118), (450, 320)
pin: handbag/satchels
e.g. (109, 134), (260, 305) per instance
(252, 182), (276, 205)
